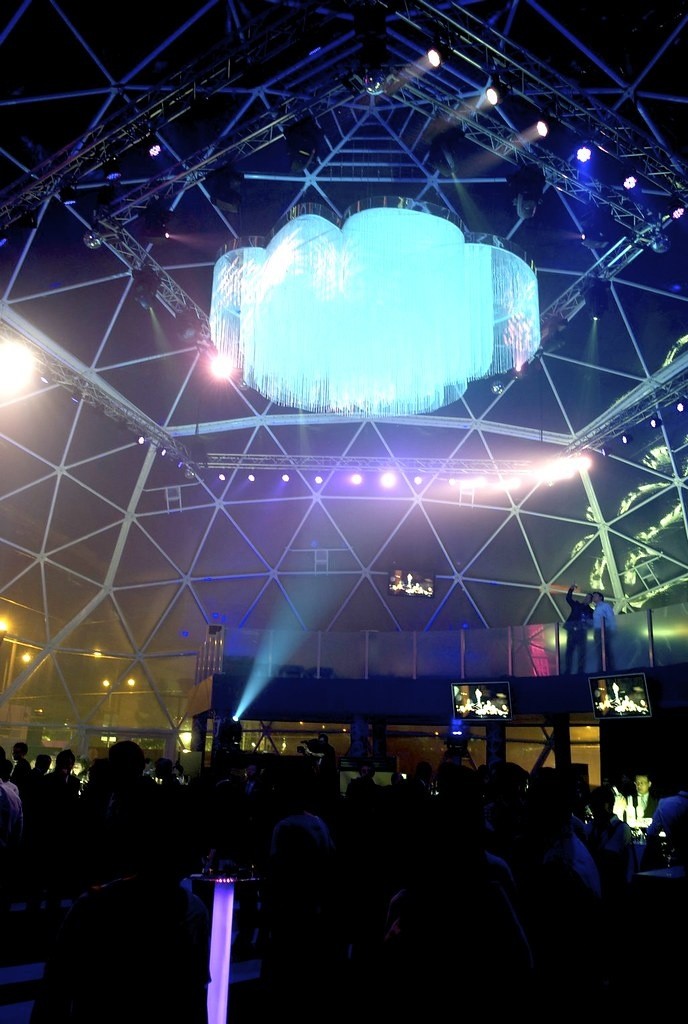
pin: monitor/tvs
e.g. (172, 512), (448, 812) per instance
(387, 565), (436, 600)
(589, 672), (653, 719)
(451, 681), (512, 720)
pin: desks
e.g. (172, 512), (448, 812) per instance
(629, 865), (688, 1024)
(189, 870), (267, 1024)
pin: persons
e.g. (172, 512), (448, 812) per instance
(0, 734), (688, 1024)
(563, 580), (594, 674)
(592, 592), (617, 673)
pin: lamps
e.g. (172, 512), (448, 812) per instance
(83, 226), (102, 250)
(135, 435), (145, 445)
(601, 446), (613, 458)
(208, 195), (540, 418)
(650, 231), (673, 255)
(103, 161), (122, 183)
(59, 185), (79, 205)
(205, 165), (245, 214)
(664, 196), (686, 222)
(537, 115), (560, 140)
(427, 42), (451, 68)
(130, 267), (161, 310)
(503, 164), (546, 219)
(69, 389), (83, 403)
(40, 370), (52, 383)
(424, 122), (466, 177)
(622, 434), (633, 445)
(486, 82), (509, 106)
(675, 400), (688, 413)
(155, 447), (167, 457)
(585, 283), (608, 324)
(649, 417), (663, 429)
(139, 201), (174, 240)
(578, 201), (614, 250)
(173, 312), (201, 343)
(281, 117), (324, 172)
